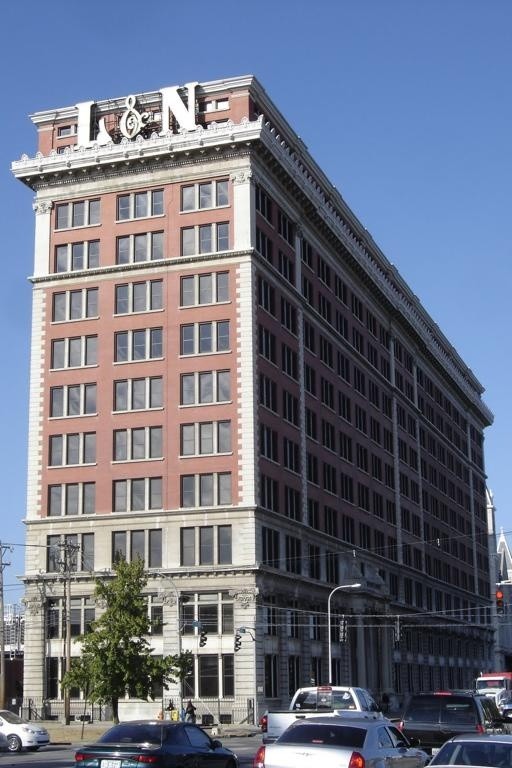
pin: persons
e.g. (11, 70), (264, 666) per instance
(185, 702), (196, 722)
(180, 708), (186, 721)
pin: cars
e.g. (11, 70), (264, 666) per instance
(0, 710), (50, 751)
(75, 721), (239, 767)
(254, 717), (430, 768)
(398, 691), (508, 749)
(424, 734), (512, 766)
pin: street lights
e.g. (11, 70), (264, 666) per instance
(143, 569), (182, 722)
(327, 583), (360, 683)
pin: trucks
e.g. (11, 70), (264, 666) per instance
(475, 672), (512, 721)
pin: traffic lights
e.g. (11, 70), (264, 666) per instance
(234, 635), (241, 652)
(200, 631), (206, 647)
(496, 590), (504, 615)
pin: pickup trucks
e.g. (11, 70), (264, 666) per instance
(262, 686), (386, 745)
